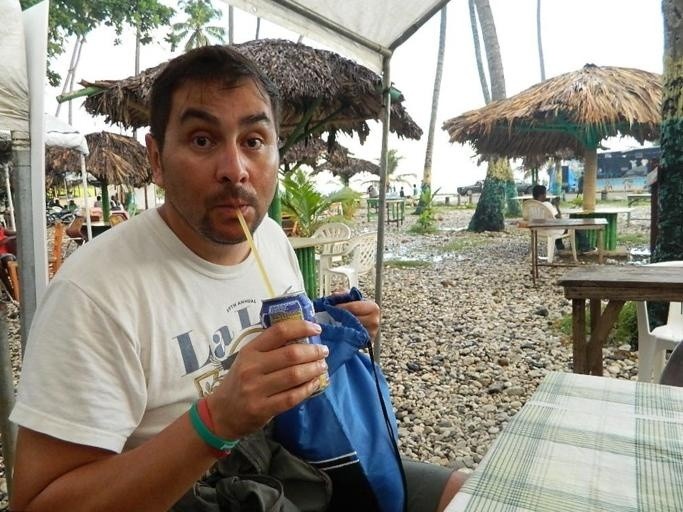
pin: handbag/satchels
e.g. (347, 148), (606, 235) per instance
(268, 288), (408, 512)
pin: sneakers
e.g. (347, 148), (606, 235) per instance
(582, 246), (598, 255)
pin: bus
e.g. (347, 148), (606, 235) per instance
(547, 146), (661, 192)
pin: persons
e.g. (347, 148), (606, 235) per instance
(7, 45), (468, 512)
(400, 186), (404, 197)
(47, 193), (124, 214)
(578, 172), (584, 193)
(413, 184), (417, 195)
(532, 185), (598, 256)
(421, 180), (423, 189)
(0, 223), (17, 269)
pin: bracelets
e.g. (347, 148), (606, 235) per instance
(190, 398), (240, 460)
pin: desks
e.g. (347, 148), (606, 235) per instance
(526, 218), (609, 285)
(444, 371), (683, 512)
(557, 265), (683, 375)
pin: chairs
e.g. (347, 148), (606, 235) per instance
(521, 199), (578, 263)
(309, 222), (378, 298)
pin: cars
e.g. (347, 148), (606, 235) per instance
(514, 179), (532, 194)
(457, 180), (485, 196)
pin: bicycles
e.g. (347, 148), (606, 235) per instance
(45, 198), (76, 228)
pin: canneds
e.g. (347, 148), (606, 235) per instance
(258, 286), (330, 400)
